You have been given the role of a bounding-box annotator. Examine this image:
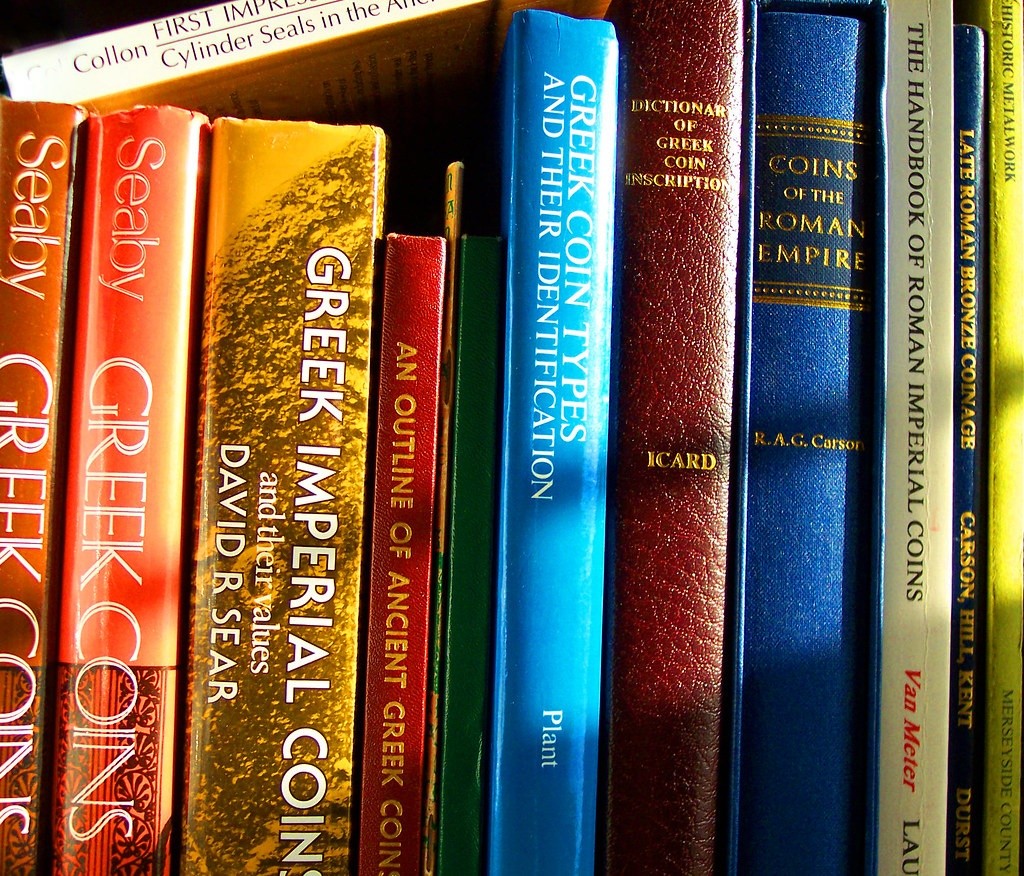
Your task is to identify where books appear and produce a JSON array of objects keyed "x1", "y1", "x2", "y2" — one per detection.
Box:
[{"x1": 2, "y1": 0, "x2": 1024, "y2": 876}]
